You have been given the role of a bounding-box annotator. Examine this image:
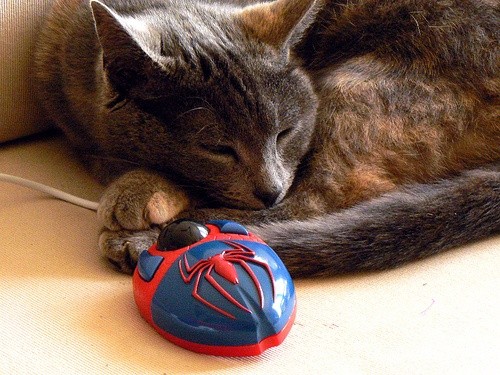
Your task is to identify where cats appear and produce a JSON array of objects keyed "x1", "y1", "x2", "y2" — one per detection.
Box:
[{"x1": 29, "y1": 0, "x2": 500, "y2": 276}]
